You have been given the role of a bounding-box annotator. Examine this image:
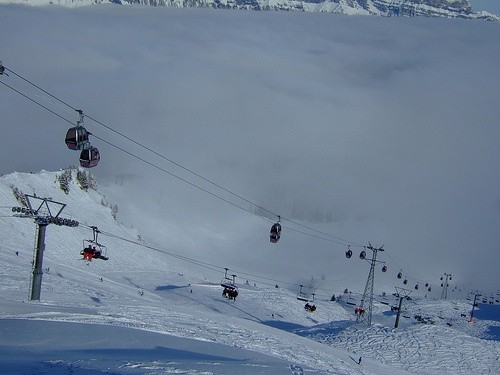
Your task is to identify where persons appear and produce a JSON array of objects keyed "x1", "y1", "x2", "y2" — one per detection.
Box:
[
  {"x1": 83, "y1": 244, "x2": 97, "y2": 262},
  {"x1": 304, "y1": 303, "x2": 316, "y2": 312},
  {"x1": 222, "y1": 288, "x2": 238, "y2": 302}
]
